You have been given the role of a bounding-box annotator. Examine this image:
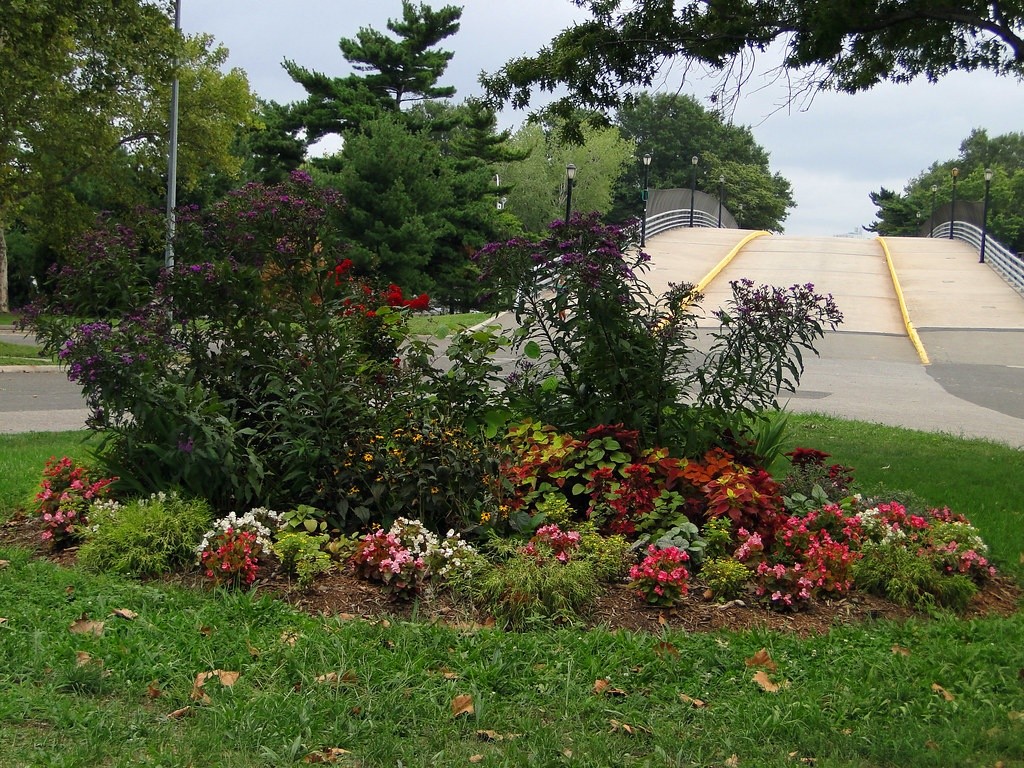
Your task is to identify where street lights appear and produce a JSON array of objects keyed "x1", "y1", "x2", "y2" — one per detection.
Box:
[
  {"x1": 738, "y1": 203, "x2": 742, "y2": 229},
  {"x1": 979, "y1": 169, "x2": 992, "y2": 263},
  {"x1": 949, "y1": 168, "x2": 960, "y2": 239},
  {"x1": 930, "y1": 184, "x2": 937, "y2": 238},
  {"x1": 718, "y1": 175, "x2": 725, "y2": 228},
  {"x1": 916, "y1": 212, "x2": 922, "y2": 236},
  {"x1": 689, "y1": 156, "x2": 698, "y2": 227},
  {"x1": 640, "y1": 153, "x2": 652, "y2": 248},
  {"x1": 565, "y1": 164, "x2": 576, "y2": 233}
]
